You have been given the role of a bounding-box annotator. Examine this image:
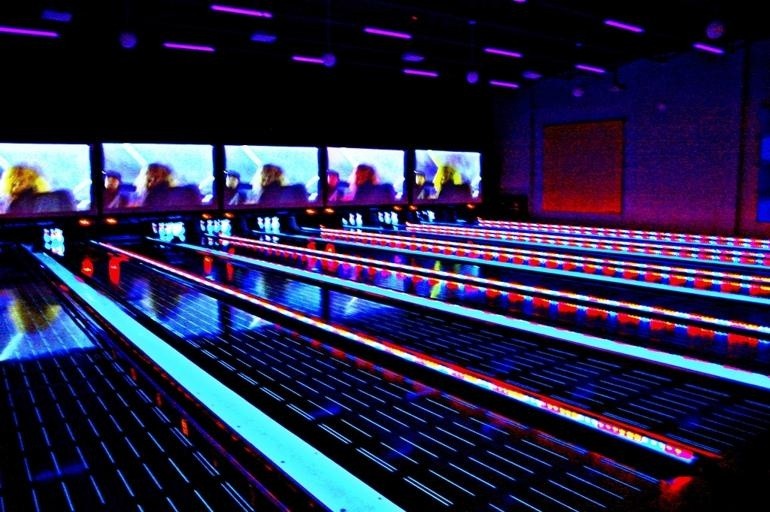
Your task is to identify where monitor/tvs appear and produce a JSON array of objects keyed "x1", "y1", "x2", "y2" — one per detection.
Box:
[
  {"x1": 326, "y1": 146, "x2": 409, "y2": 207},
  {"x1": 412, "y1": 149, "x2": 483, "y2": 205},
  {"x1": 223, "y1": 144, "x2": 324, "y2": 210},
  {"x1": 101, "y1": 143, "x2": 219, "y2": 214},
  {"x1": 0, "y1": 142, "x2": 98, "y2": 219}
]
